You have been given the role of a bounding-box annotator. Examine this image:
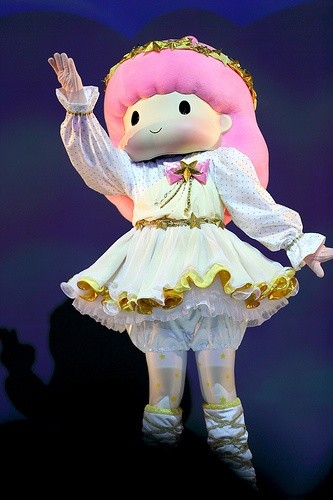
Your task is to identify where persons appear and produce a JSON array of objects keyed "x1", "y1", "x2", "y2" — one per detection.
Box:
[{"x1": 48, "y1": 36, "x2": 333, "y2": 491}]
